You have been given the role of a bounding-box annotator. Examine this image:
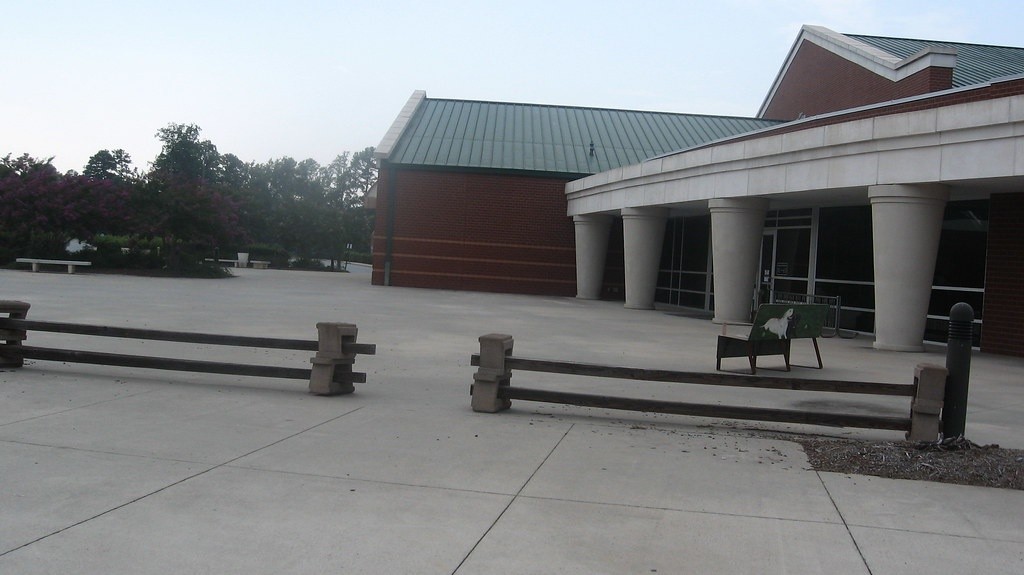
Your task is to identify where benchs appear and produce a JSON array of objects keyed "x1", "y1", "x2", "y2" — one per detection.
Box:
[
  {"x1": 15, "y1": 258, "x2": 91, "y2": 274},
  {"x1": 204, "y1": 258, "x2": 239, "y2": 267},
  {"x1": 716, "y1": 303, "x2": 829, "y2": 375},
  {"x1": 250, "y1": 260, "x2": 271, "y2": 269}
]
